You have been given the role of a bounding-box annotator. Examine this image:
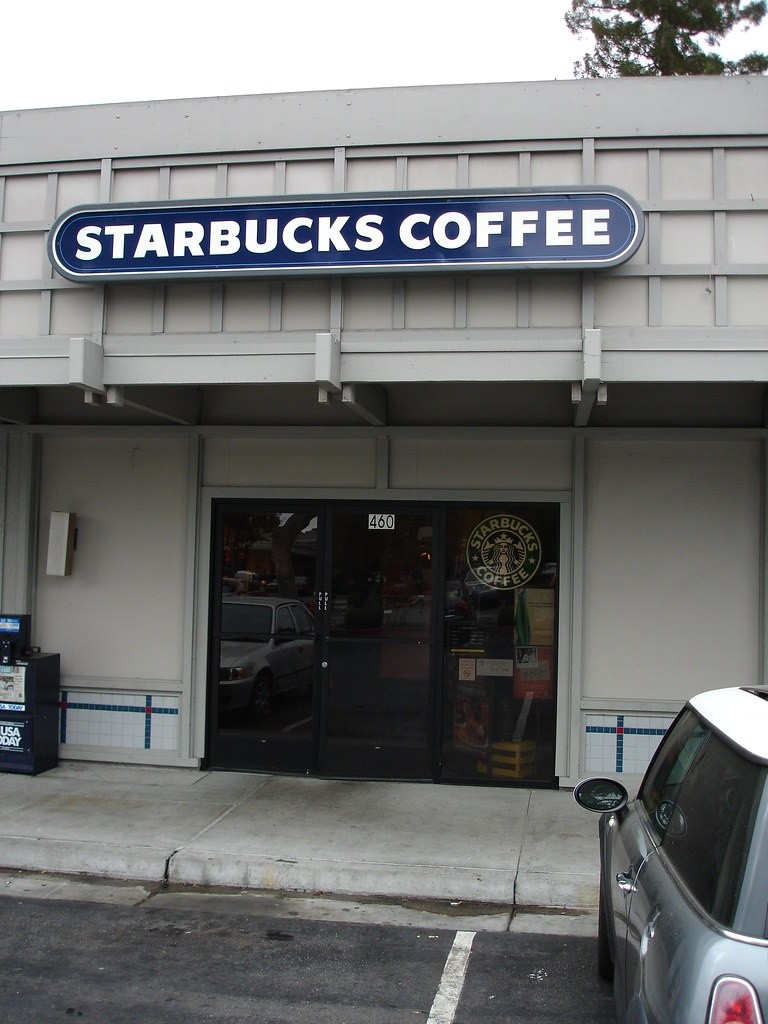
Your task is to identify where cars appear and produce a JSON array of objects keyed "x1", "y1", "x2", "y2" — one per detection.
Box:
[
  {"x1": 223, "y1": 570, "x2": 314, "y2": 597},
  {"x1": 222, "y1": 597, "x2": 317, "y2": 721},
  {"x1": 574, "y1": 689, "x2": 768, "y2": 1024},
  {"x1": 463, "y1": 568, "x2": 505, "y2": 608}
]
